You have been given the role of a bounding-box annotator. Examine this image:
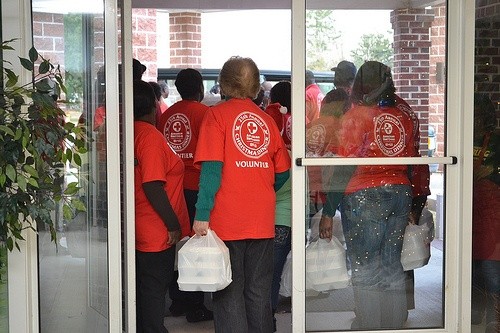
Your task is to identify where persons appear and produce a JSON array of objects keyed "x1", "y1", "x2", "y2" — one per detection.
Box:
[
  {"x1": 472, "y1": 150, "x2": 500, "y2": 293},
  {"x1": 28, "y1": 56, "x2": 431, "y2": 333}
]
